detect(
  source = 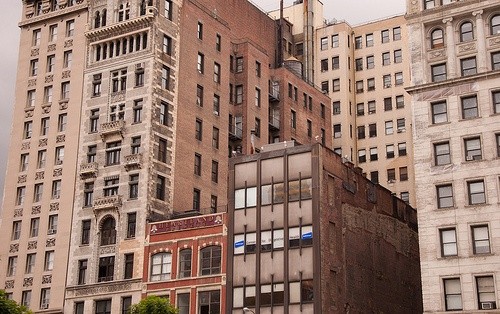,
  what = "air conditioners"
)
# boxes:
[47,229,54,234]
[482,303,493,310]
[101,277,106,282]
[40,303,48,308]
[397,129,402,133]
[466,156,473,160]
